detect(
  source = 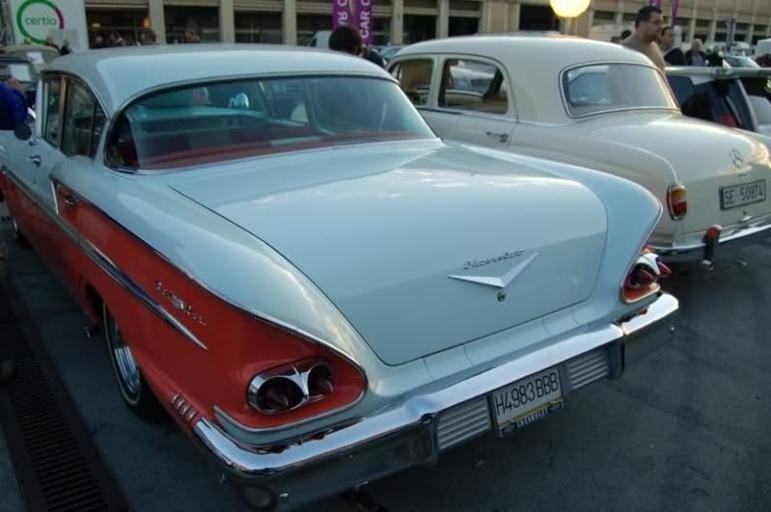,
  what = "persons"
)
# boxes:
[184,29,201,42]
[328,25,384,69]
[91,16,157,49]
[0,77,32,140]
[611,5,723,72]
[46,35,72,55]
